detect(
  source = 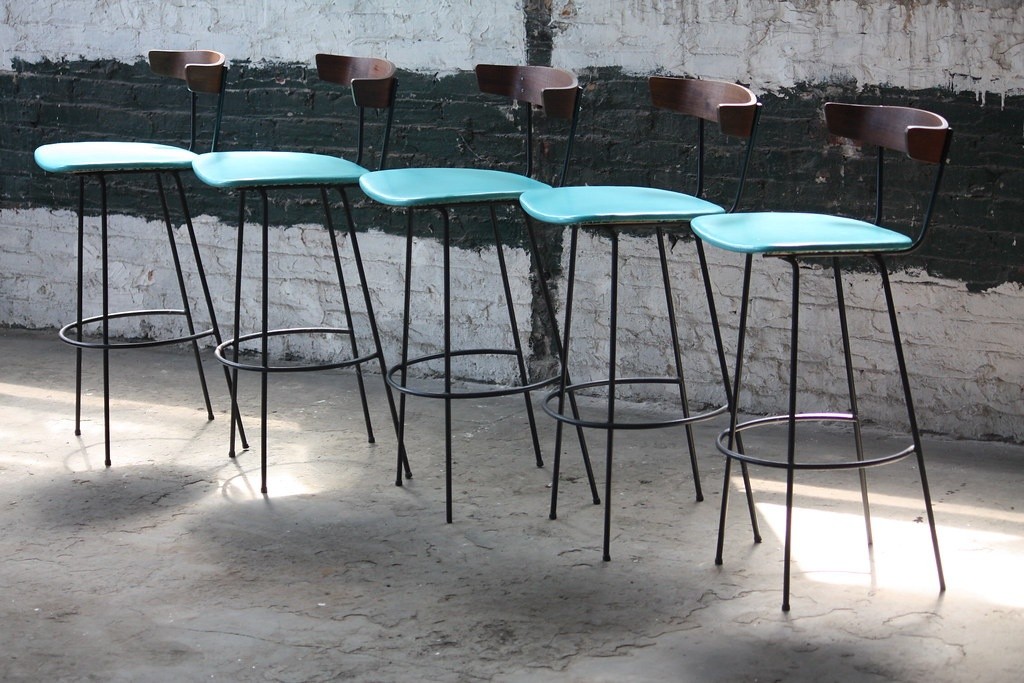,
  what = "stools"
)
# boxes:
[360,63,586,526]
[518,75,764,561]
[687,102,954,610]
[191,53,414,493]
[33,49,248,467]
